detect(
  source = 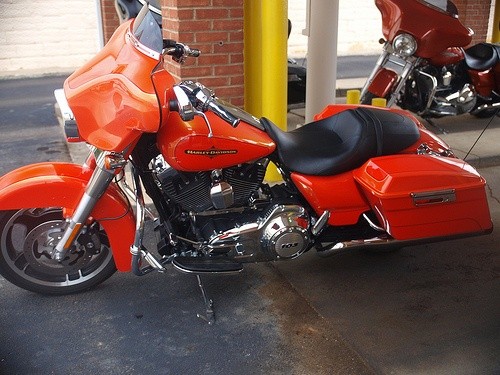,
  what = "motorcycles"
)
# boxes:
[360,0,500,135]
[0,1,495,329]
